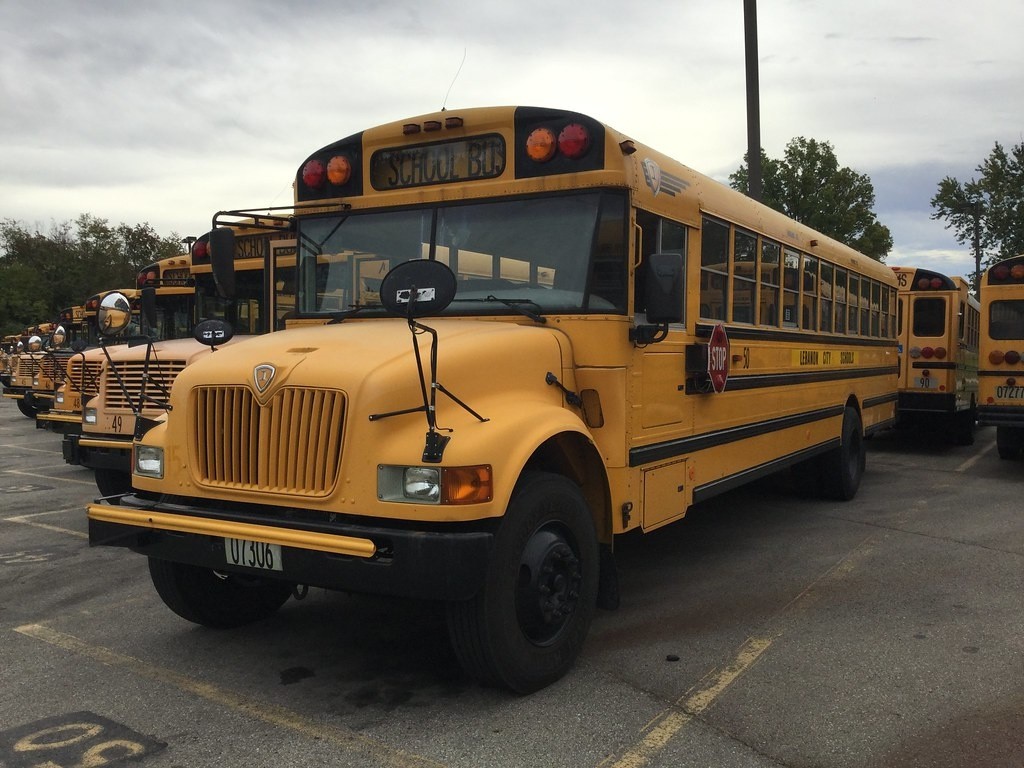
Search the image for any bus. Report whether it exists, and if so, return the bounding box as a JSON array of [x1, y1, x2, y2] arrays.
[[976, 250, 1023, 474], [884, 266, 982, 448], [16, 101, 906, 704]]
